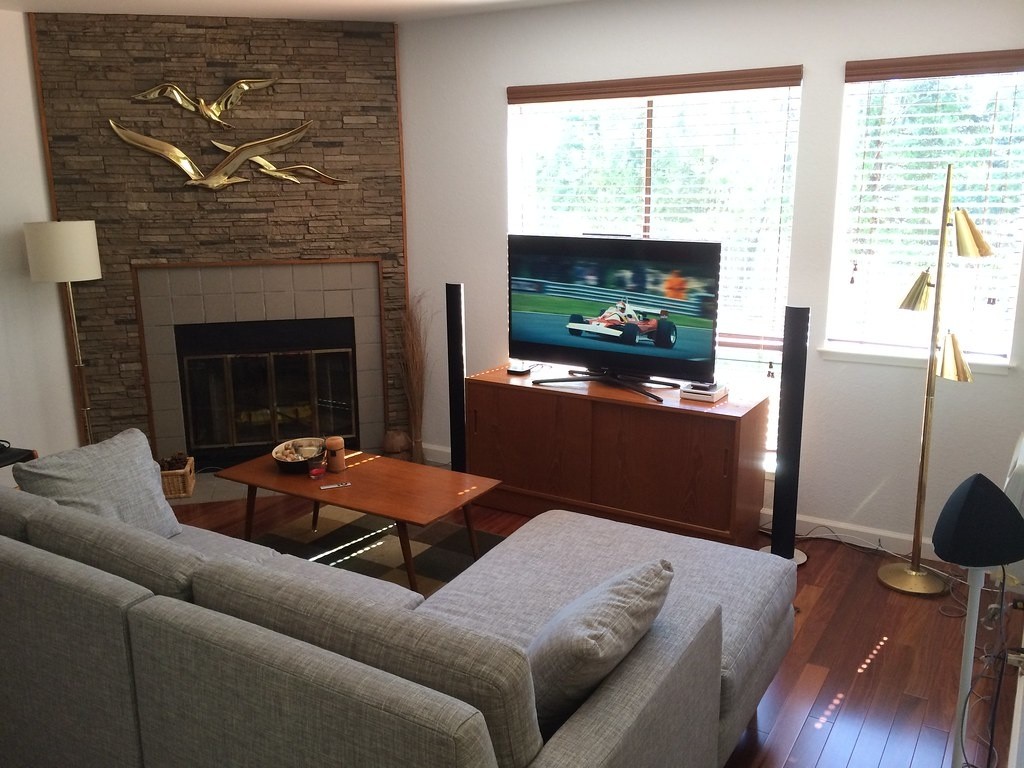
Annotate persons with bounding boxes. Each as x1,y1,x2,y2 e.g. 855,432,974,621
665,269,687,300
615,301,626,314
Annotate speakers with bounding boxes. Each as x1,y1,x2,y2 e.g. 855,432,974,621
445,282,466,472
759,305,810,564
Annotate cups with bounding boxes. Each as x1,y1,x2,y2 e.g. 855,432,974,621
308,457,325,479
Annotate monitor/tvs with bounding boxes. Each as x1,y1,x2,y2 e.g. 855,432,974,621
508,234,720,402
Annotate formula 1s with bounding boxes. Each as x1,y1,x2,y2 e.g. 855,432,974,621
568,297,678,350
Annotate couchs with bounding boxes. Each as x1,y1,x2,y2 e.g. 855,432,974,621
0,426,800,768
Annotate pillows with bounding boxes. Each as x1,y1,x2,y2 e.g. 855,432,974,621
525,558,675,745
11,428,183,538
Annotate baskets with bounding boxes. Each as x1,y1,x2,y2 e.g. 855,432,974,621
161,457,196,498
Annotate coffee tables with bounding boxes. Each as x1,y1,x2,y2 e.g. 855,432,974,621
213,448,502,596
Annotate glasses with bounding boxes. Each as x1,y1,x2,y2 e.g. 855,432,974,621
616,306,625,310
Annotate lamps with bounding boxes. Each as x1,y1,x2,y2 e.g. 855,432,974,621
876,163,994,599
24,219,102,447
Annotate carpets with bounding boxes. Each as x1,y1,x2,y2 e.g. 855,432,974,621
249,505,506,598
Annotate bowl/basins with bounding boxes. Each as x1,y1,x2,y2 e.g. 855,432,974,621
272,437,326,475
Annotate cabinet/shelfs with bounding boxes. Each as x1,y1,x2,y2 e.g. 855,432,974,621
463,361,771,549
